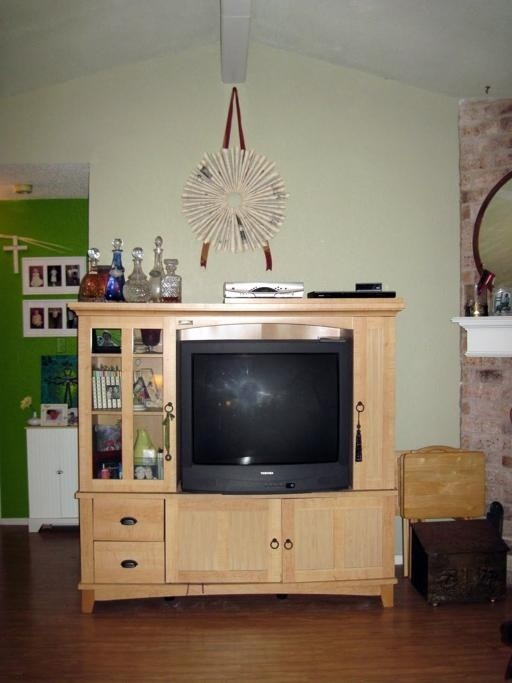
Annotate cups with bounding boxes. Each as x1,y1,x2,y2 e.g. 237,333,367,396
94,461,122,479
135,339,150,354
465,283,487,316
134,404,145,409
490,287,512,315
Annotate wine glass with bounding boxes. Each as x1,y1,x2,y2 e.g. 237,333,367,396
141,329,160,353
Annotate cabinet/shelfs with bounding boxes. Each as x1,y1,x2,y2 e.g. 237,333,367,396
68,295,406,613
24,427,81,534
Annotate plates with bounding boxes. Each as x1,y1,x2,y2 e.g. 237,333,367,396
133,408,146,410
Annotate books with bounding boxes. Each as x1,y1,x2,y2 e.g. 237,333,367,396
92,369,121,410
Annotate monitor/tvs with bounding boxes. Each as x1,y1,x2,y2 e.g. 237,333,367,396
177,339,353,493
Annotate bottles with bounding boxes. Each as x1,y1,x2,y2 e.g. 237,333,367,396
79,233,182,304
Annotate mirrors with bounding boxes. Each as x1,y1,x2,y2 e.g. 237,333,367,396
471,169,512,294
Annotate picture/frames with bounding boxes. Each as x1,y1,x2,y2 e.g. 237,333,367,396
40,403,69,427
19,254,88,339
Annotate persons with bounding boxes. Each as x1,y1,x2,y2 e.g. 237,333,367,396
31,309,43,327
136,466,145,479
147,467,155,479
67,265,78,276
68,310,76,328
69,271,80,285
47,409,61,418
102,331,114,345
52,308,60,327
51,267,61,286
31,266,43,286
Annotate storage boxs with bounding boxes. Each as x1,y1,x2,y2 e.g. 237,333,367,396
406,518,511,607
